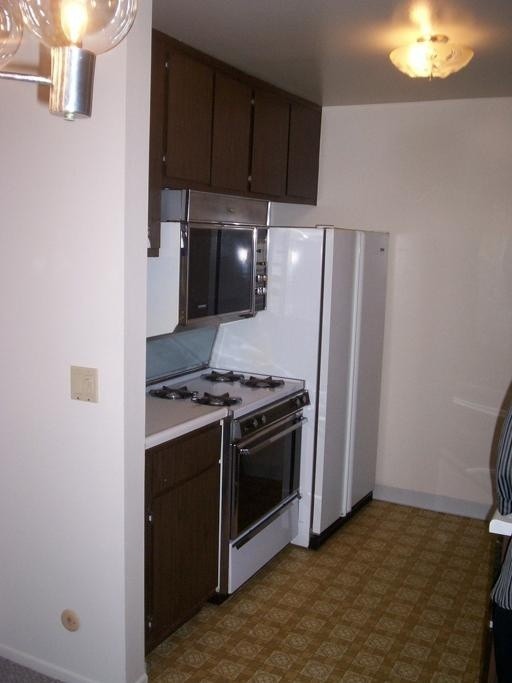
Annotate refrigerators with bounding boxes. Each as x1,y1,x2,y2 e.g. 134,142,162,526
212,226,388,550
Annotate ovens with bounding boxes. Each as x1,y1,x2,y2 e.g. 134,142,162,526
223,409,309,602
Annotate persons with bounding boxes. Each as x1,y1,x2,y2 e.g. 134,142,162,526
487,409,512,683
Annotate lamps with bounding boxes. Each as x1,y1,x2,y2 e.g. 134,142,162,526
390,36,475,81
0,1,140,124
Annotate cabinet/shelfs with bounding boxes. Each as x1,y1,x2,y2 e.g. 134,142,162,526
145,419,222,655
147,28,322,260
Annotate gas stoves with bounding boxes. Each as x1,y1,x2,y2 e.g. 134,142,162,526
149,362,310,442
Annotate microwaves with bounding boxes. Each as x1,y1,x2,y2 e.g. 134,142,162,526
146,187,268,342
